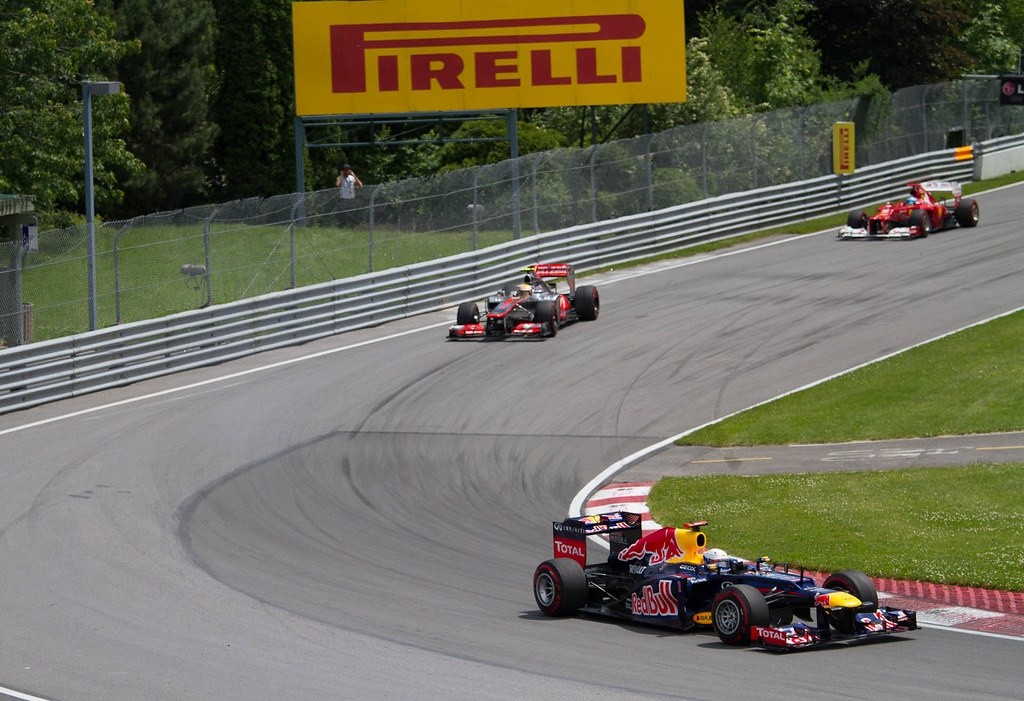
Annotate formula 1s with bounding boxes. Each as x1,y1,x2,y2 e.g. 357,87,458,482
445,263,600,342
836,180,978,242
533,508,920,654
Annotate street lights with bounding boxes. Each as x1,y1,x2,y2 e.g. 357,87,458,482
81,79,119,334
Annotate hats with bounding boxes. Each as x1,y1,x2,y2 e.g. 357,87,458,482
341,163,351,171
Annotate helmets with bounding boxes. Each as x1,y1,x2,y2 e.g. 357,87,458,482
519,284,532,292
907,197,918,202
703,548,731,573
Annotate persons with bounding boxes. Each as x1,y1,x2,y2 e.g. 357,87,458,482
906,187,925,205
703,549,730,573
517,284,531,300
335,164,363,229
